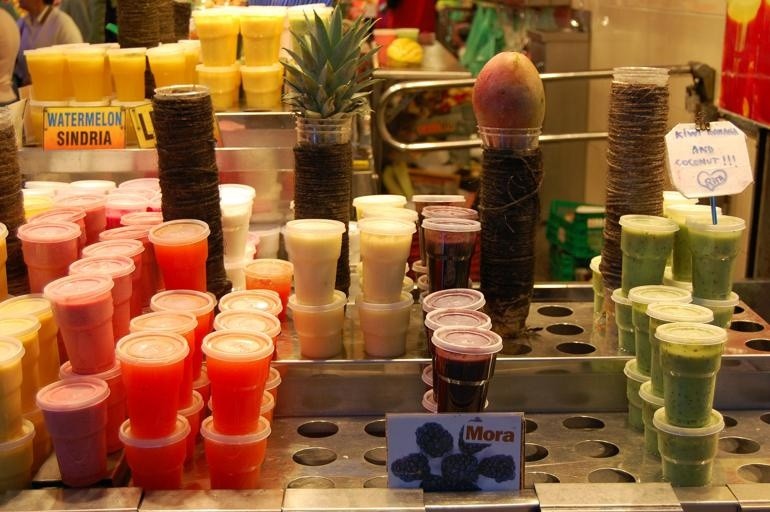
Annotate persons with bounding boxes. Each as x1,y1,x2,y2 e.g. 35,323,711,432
374,1,436,31
13,0,82,87
0,7,21,105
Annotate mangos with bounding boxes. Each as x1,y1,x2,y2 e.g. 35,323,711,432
471,51,547,150
